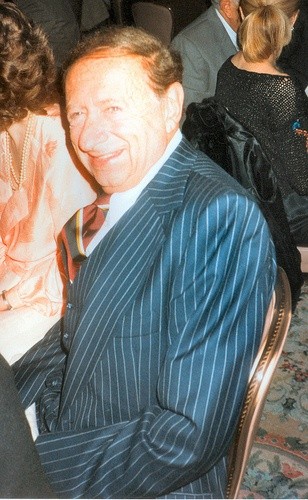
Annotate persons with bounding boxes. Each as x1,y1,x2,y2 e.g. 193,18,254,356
8,28,278,499
12,0,82,69
0,0,102,367
0,355,59,499
172,0,308,247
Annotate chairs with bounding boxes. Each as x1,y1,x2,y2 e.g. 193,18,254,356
185,99,303,310
130,0,176,52
224,264,293,499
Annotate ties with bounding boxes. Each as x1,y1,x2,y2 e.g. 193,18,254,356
60,193,111,317
